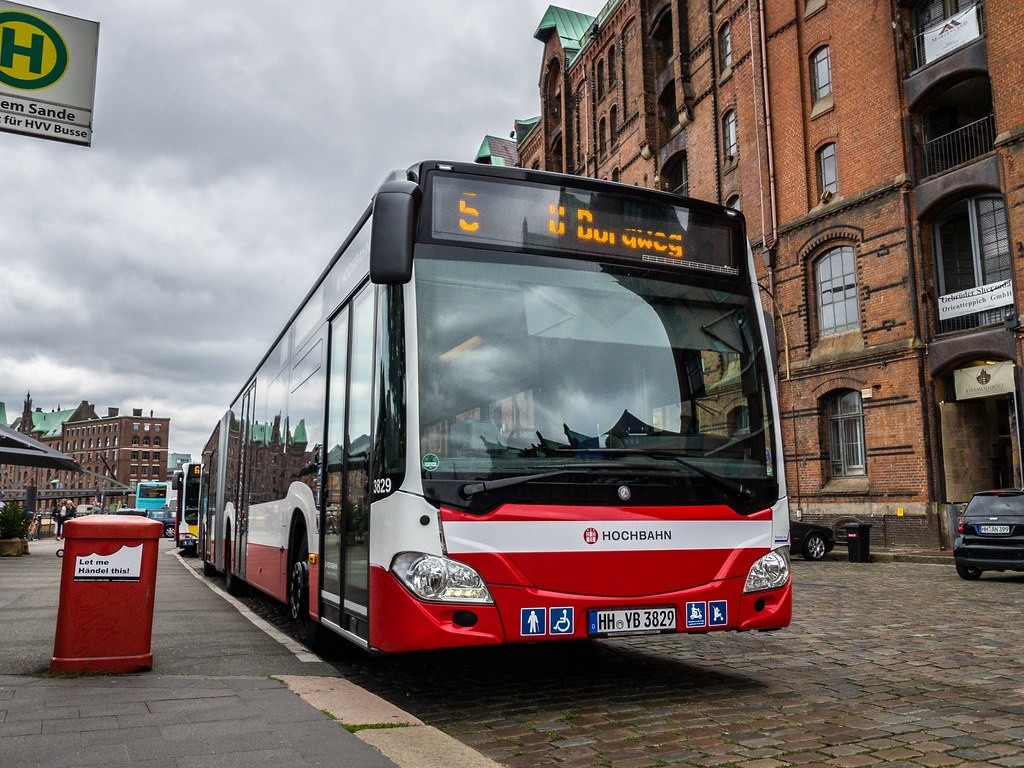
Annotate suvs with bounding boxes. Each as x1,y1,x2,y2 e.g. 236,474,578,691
953,488,1024,581
147,510,178,538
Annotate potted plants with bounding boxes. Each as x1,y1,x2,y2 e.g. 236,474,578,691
0,498,32,556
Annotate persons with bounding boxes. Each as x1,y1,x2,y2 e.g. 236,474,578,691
51,499,76,540
161,503,169,509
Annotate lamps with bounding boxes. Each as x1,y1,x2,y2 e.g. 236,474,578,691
510,131,517,139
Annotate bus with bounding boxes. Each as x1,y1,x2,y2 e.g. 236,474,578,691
135,480,178,509
198,161,791,655
172,462,201,552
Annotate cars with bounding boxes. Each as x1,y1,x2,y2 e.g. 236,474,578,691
789,517,837,562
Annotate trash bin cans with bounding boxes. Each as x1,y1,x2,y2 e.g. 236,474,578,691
845,522,872,563
116,508,149,518
49,515,163,678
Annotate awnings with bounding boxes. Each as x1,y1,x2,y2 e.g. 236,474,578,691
0,422,83,474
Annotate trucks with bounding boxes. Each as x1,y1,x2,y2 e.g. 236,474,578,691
169,497,193,518
